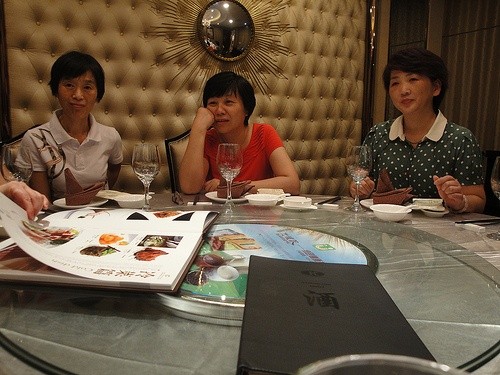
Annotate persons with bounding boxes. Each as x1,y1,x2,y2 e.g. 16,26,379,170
178,71,301,195
349,48,486,213
0,164,50,220
14,50,124,198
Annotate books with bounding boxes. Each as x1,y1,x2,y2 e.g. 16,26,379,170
0,192,220,295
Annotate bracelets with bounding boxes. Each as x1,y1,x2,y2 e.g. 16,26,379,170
445,195,468,213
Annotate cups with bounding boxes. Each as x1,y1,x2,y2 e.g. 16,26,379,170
194,253,250,282
2,146,34,183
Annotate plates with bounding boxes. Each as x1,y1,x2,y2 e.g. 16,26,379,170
360,199,412,209
421,209,449,217
205,191,248,203
52,198,108,209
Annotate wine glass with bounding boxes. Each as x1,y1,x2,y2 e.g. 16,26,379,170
131,144,161,212
344,146,372,214
216,144,244,208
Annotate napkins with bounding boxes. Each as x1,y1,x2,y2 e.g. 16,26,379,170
372,166,415,205
216,177,255,198
64,168,105,206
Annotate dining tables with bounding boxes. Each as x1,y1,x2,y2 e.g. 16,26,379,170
0,192,500,375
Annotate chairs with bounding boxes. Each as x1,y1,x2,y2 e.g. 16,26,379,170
164,129,192,192
1,123,42,175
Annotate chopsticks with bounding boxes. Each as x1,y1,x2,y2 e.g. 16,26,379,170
454,219,500,224
318,196,341,205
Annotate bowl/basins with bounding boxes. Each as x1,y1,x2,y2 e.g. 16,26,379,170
370,204,411,221
245,194,283,207
114,194,153,208
